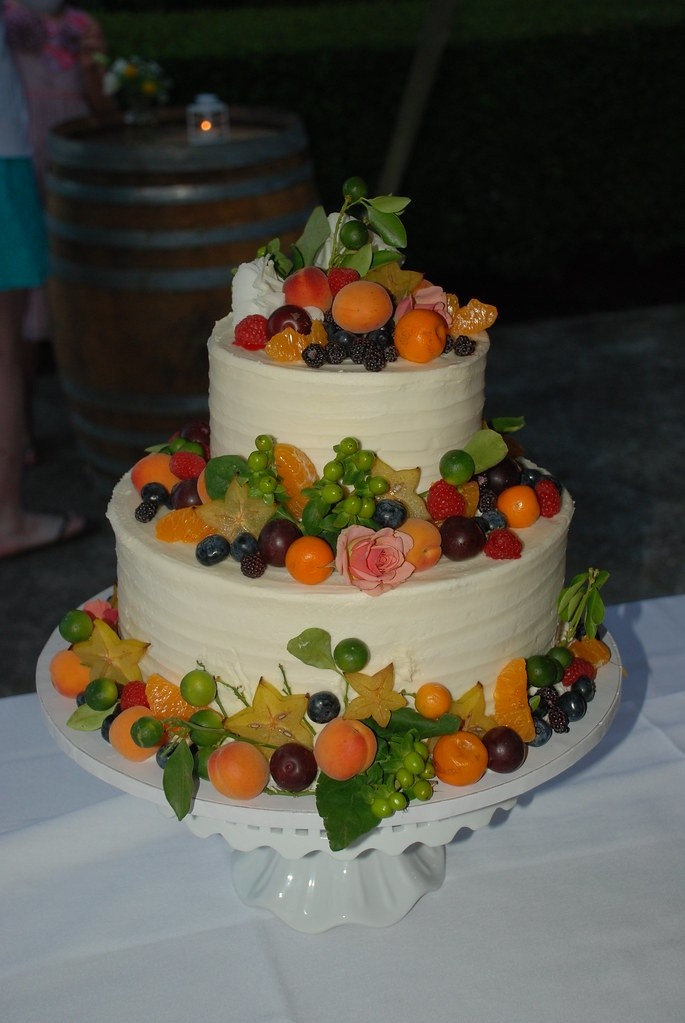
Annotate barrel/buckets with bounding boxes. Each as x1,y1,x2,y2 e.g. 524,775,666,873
47,106,319,539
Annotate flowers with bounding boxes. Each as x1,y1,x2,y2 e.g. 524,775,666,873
392,286,453,328
317,525,415,596
292,178,410,277
231,238,292,325
102,55,172,108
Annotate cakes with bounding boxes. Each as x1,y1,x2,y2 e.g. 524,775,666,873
37,181,609,850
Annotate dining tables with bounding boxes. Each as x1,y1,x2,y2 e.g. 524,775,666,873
0,591,684,1023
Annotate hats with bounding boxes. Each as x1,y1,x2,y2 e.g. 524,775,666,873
62,7,104,44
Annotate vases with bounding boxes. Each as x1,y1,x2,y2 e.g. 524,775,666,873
123,95,159,126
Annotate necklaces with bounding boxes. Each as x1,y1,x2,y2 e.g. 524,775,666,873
0,0,98,567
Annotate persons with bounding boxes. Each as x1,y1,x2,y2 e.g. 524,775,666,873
5,0,109,468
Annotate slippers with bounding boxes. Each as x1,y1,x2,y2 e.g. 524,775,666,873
1,511,98,562
